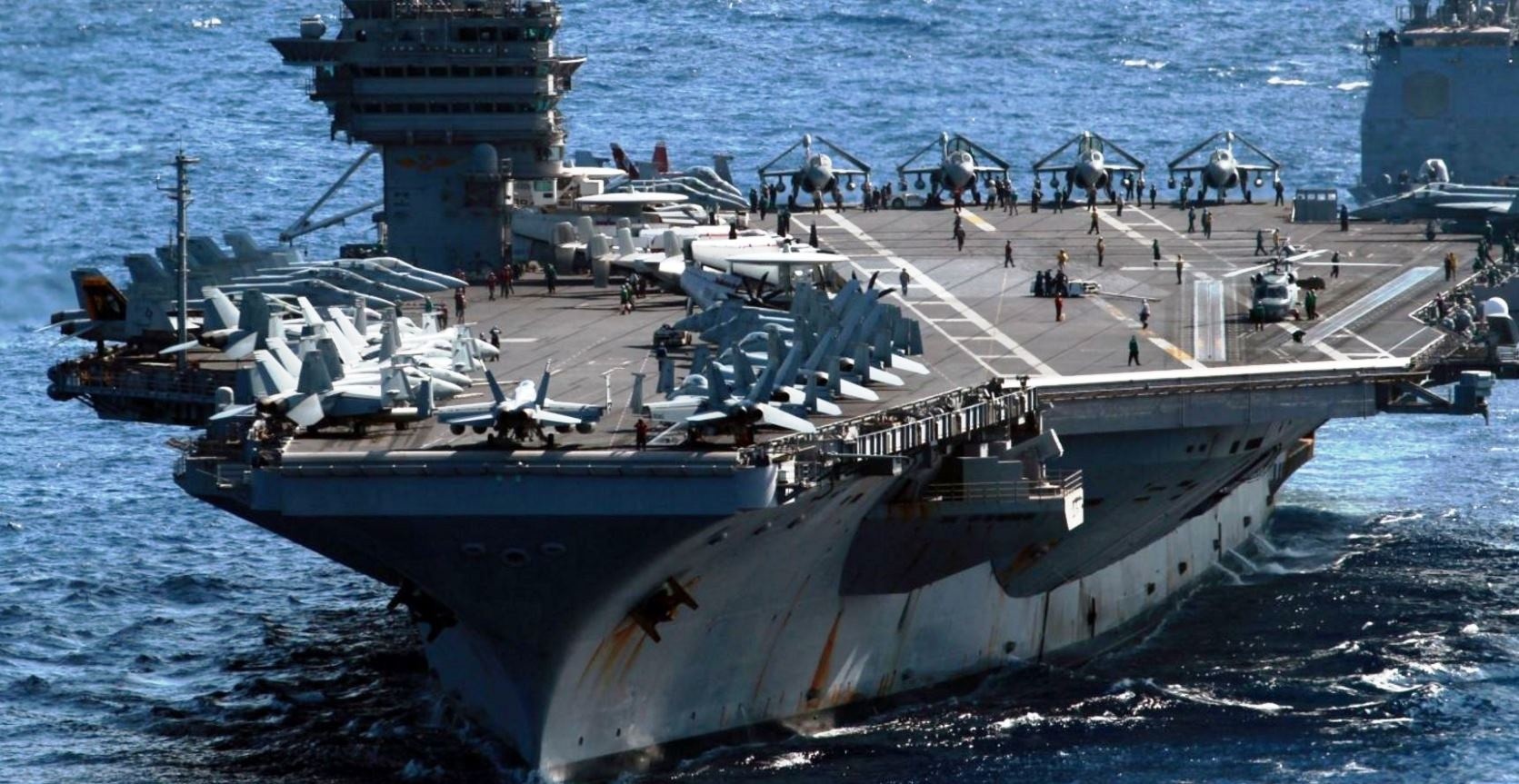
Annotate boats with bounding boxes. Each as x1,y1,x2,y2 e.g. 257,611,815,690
539,56,587,78
45,0,1519,783
267,17,357,62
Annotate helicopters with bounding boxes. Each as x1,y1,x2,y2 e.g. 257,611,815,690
1221,249,1403,321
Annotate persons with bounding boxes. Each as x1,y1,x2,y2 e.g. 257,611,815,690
394,262,558,362
618,271,647,315
1088,207,1105,267
1253,227,1281,257
1034,247,1070,321
656,341,668,371
1434,220,1519,333
1330,250,1339,279
1004,240,1015,268
952,189,966,251
634,418,646,451
1153,206,1212,285
1339,203,1349,231
1275,182,1285,206
1128,298,1151,367
749,181,893,248
1306,289,1320,320
983,172,1157,217
899,268,910,296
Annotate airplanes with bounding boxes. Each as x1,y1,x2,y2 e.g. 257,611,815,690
628,264,930,434
604,142,751,211
1033,131,1146,204
598,226,849,285
431,359,611,448
1348,182,1519,242
1167,130,1281,204
35,230,470,354
209,296,502,439
897,132,1011,209
756,134,871,210
